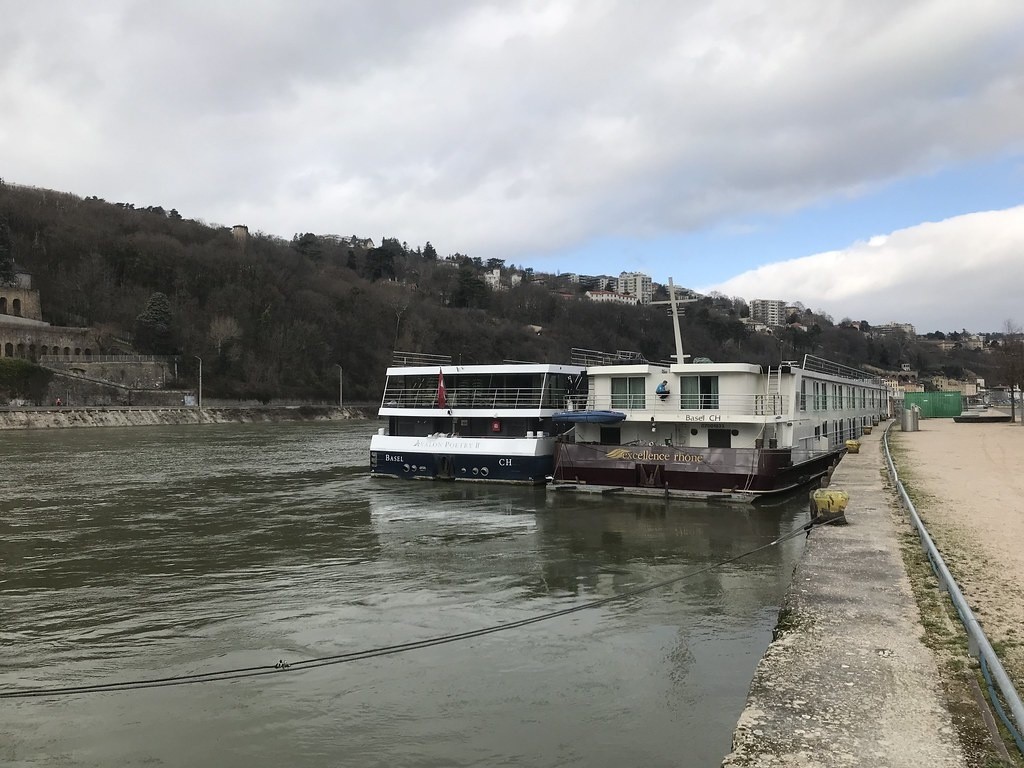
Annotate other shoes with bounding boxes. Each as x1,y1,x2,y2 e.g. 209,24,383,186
660,397,665,401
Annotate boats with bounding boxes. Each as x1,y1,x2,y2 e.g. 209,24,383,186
552,411,627,424
370,351,589,486
552,274,892,504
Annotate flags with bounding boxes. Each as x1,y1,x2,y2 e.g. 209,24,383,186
438,369,445,409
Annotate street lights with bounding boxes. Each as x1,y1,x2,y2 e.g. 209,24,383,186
195,355,201,406
335,365,342,405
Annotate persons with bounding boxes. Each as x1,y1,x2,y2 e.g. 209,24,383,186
567,375,575,410
656,380,670,401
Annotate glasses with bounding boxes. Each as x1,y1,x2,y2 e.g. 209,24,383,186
665,384,666,385
